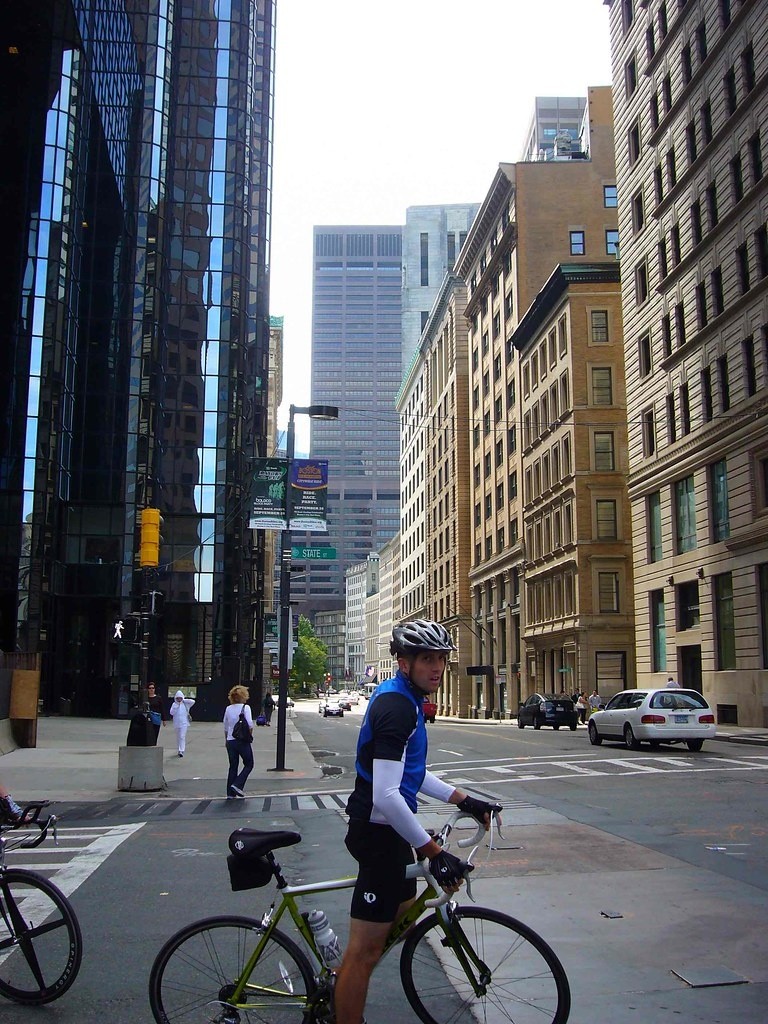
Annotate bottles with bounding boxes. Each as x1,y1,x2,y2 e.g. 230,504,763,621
308,909,344,969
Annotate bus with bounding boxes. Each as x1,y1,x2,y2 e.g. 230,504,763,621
365,683,379,700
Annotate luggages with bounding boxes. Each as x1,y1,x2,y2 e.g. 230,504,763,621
256,712,266,726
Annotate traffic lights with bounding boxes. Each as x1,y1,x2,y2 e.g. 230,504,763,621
328,678,330,680
110,617,139,643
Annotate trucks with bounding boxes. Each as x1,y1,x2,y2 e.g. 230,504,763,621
359,689,365,696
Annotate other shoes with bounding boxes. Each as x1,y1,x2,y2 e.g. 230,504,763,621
178,752,184,756
230,785,245,797
227,795,234,800
266,724,270,726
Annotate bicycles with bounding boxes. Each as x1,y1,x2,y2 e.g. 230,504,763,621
0,799,84,1005
148,795,573,1024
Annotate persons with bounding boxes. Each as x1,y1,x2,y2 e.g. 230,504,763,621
170,690,195,757
223,685,254,800
144,682,166,746
332,617,502,1024
0,785,31,828
560,688,601,725
263,693,275,727
666,677,680,688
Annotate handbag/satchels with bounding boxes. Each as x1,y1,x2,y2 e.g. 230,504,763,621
127,710,161,747
575,696,586,709
232,704,253,746
183,701,192,722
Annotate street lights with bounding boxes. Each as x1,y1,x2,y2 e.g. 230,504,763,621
323,672,330,697
273,401,339,769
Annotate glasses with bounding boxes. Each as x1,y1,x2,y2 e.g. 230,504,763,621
149,687,154,690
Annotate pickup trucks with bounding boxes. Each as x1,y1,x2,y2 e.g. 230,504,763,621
421,697,437,723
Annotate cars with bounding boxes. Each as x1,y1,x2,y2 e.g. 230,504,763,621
318,688,365,705
318,700,343,718
272,695,279,706
337,699,351,711
588,688,716,751
517,692,579,730
286,697,294,708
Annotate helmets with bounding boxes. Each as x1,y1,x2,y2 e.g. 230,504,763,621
389,619,458,652
